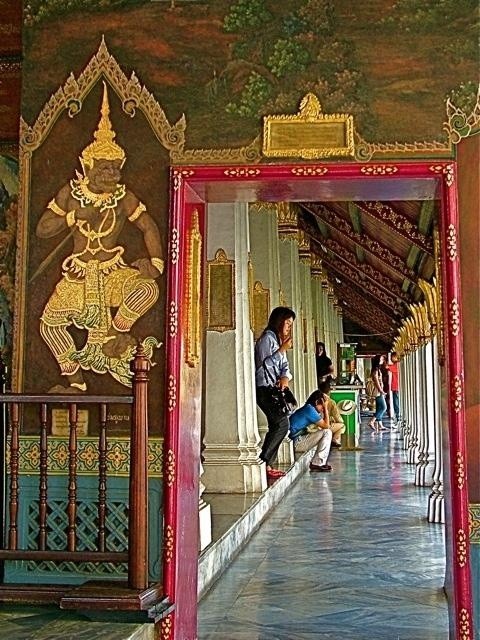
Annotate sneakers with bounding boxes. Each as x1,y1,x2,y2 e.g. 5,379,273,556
331,441,341,447
310,462,332,472
267,470,285,479
367,422,376,430
378,427,388,430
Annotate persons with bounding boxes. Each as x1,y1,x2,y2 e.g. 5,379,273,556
367,353,389,432
34,139,165,394
383,352,400,429
320,381,346,450
316,341,335,396
254,306,296,479
287,389,333,472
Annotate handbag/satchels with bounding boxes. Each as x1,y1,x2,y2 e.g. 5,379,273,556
366,382,380,397
268,385,297,415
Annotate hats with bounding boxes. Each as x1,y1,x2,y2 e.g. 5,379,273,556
336,400,356,415
